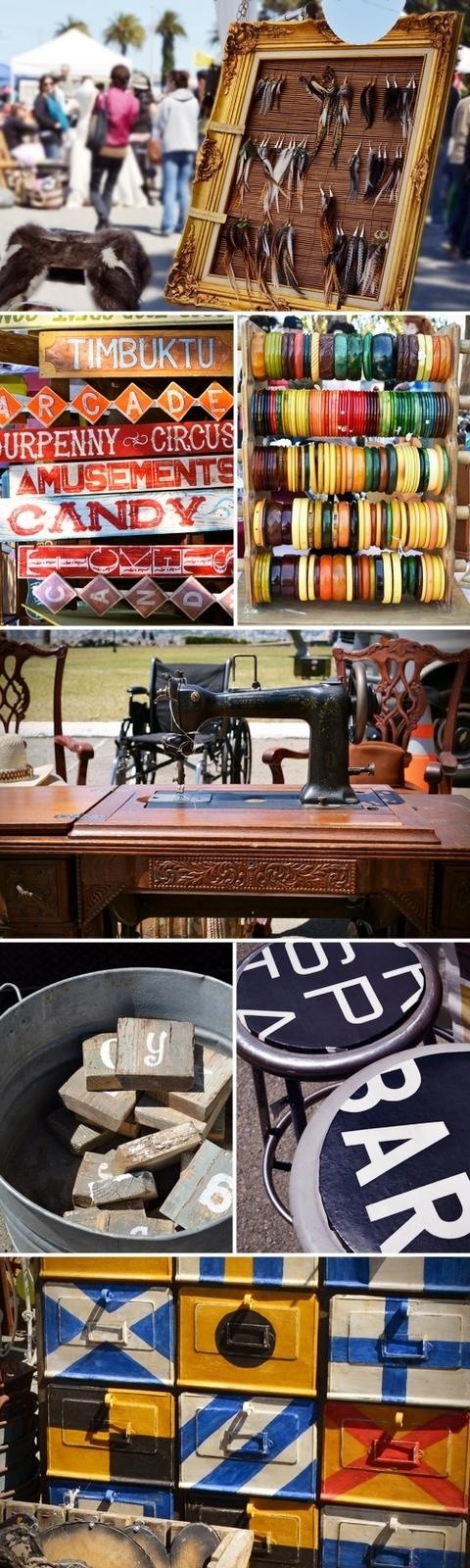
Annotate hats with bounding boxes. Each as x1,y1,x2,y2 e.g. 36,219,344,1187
0,733,55,787
131,75,150,90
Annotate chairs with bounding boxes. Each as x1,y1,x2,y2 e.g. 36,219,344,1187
0,629,94,787
261,638,470,794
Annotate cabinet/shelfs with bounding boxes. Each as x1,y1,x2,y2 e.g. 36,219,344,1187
239,318,470,613
34,1257,470,1567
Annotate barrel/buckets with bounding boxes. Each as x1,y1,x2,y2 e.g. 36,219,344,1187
0,966,232,1252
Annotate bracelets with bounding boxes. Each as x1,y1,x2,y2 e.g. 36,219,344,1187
249,331,453,606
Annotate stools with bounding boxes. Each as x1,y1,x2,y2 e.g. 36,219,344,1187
230,937,445,1228
287,1039,469,1257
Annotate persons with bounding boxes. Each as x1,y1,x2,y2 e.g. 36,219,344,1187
0,63,203,237
425,71,470,263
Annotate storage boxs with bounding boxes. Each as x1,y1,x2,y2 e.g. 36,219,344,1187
1,1500,257,1567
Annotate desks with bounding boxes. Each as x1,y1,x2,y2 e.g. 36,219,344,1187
0,786,469,982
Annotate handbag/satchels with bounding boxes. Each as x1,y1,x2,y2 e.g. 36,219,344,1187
349,741,411,788
147,141,161,165
85,110,108,154
23,172,64,209
